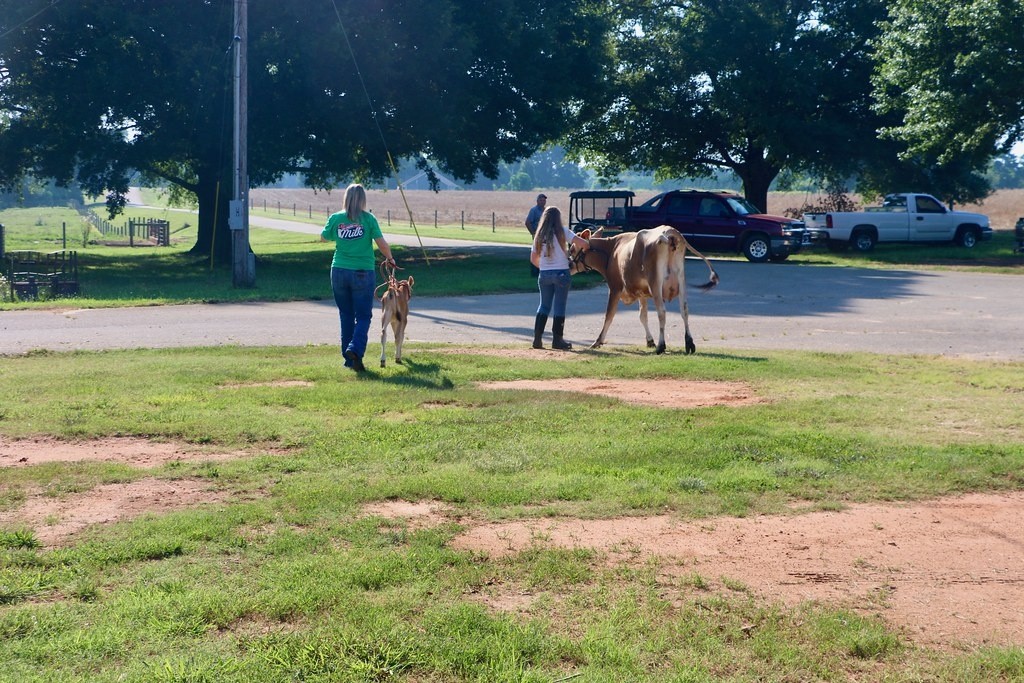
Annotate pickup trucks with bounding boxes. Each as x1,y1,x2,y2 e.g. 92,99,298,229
799,192,994,253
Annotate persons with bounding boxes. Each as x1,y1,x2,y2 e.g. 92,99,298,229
525,194,547,278
321,182,394,371
530,206,589,350
1015,218,1024,253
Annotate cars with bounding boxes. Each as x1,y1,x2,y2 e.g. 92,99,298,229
605,189,813,264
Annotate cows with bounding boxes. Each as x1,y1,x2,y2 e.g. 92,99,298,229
569,225,720,356
379,275,415,367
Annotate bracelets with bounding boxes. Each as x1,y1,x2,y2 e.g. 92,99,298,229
386,257,393,262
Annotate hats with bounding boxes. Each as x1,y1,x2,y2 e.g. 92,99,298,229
537,194,547,201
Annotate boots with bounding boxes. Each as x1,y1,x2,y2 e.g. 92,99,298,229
533,314,548,347
552,315,572,348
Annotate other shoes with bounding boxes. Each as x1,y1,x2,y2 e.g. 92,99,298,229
343,348,365,372
